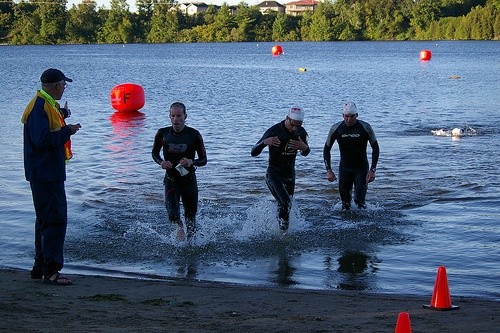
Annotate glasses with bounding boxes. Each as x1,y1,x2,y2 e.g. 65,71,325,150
289,118,301,128
345,114,355,118
58,83,67,88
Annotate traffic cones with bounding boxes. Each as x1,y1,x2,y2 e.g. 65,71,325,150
394,311,412,333
422,266,460,312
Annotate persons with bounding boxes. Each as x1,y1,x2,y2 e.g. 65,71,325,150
251,106,311,236
152,103,207,240
22,68,81,286
323,101,380,212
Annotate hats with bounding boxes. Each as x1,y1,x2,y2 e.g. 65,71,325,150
41,68,73,83
343,101,357,114
288,107,305,121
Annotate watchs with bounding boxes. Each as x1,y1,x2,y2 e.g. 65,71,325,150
371,168,376,173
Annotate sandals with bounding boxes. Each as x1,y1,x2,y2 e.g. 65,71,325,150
42,272,74,286
31,272,42,278
